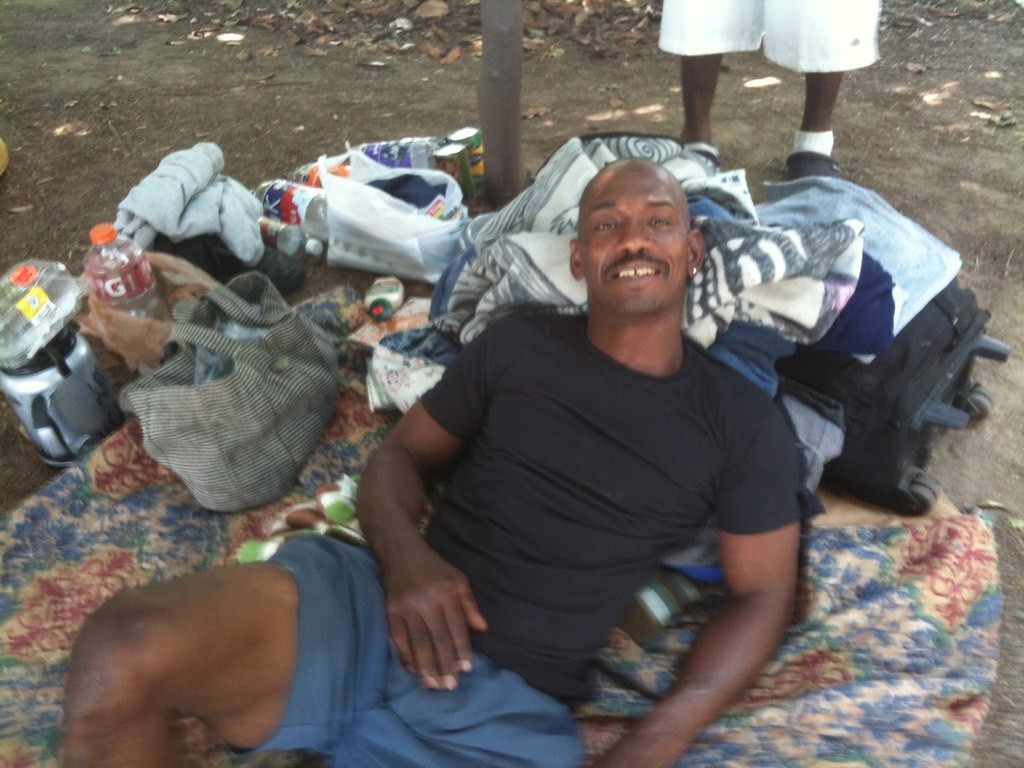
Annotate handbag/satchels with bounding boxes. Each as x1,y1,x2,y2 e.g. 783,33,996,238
88,249,341,513
316,141,470,284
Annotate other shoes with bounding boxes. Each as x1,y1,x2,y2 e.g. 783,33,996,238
786,151,842,181
682,140,723,167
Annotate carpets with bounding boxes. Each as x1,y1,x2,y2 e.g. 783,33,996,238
0,297,1001,768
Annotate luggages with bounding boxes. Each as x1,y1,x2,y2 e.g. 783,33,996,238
786,277,1013,518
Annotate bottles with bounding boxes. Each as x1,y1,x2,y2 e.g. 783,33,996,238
84,222,175,369
244,125,485,259
364,275,404,320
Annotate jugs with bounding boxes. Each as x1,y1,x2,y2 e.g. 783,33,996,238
0,321,123,467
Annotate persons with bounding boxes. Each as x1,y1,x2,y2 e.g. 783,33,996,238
658,0,885,180
62,159,826,768
